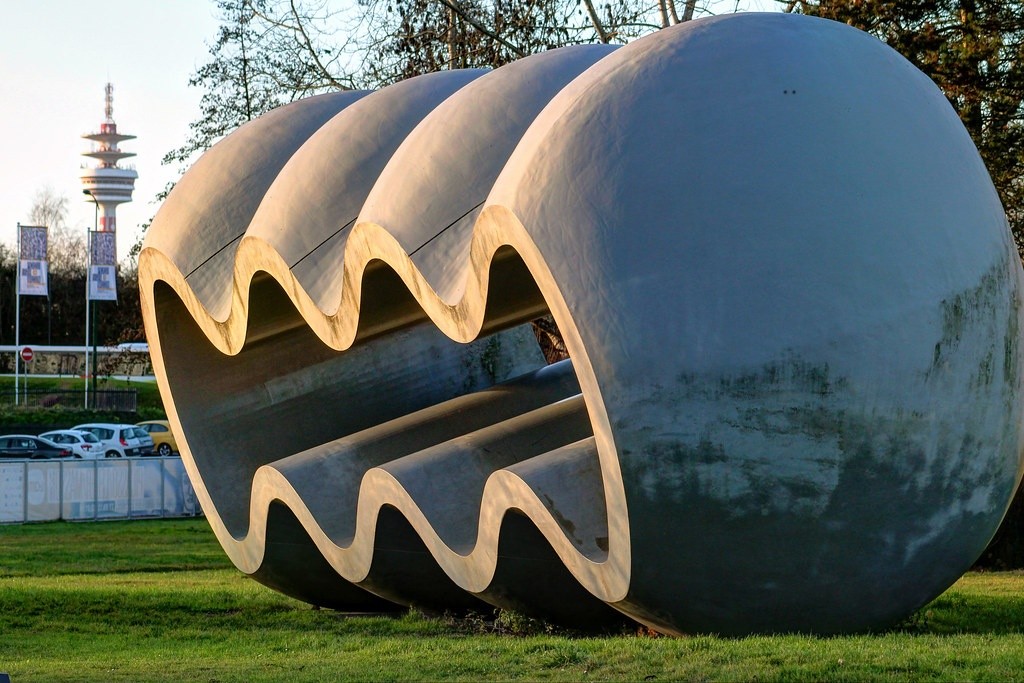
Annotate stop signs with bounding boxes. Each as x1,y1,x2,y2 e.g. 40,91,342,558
21,348,35,362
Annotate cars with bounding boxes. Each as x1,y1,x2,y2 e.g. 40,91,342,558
38,430,105,459
0,435,76,462
135,418,180,457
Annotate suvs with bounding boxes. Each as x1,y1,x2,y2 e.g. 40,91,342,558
71,423,156,458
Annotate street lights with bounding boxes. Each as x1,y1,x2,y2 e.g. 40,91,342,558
81,188,100,410
